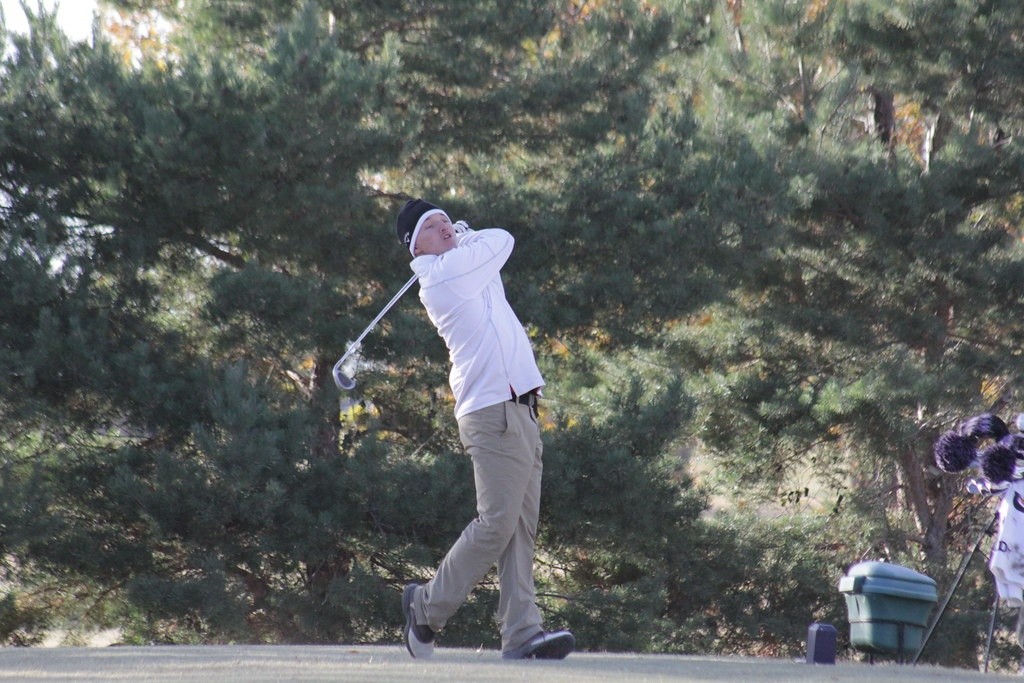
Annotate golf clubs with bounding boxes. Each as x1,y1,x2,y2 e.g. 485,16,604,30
331,220,469,391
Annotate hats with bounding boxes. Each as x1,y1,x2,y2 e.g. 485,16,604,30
396,200,451,259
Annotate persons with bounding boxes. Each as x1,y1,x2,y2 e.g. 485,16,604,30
394,199,577,661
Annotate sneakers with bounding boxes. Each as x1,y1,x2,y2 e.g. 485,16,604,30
402,583,434,659
504,629,574,660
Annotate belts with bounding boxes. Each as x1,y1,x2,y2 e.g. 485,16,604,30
509,393,536,409
